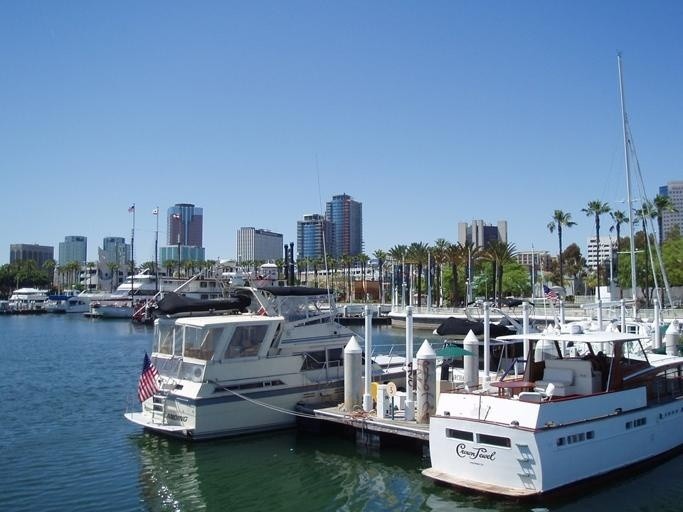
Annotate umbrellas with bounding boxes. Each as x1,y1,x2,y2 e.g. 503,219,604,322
430,344,476,382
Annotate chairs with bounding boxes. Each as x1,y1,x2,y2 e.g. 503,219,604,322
519,392,541,404
482,376,503,394
539,382,555,402
464,384,486,395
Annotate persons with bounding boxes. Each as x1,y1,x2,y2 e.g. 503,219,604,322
592,350,609,372
435,359,451,381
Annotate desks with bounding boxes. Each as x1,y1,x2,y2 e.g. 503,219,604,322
489,381,536,398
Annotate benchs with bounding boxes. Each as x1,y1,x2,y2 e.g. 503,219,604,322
544,359,602,394
533,368,577,397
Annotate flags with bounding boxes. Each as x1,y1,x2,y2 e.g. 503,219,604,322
126,205,134,214
136,355,160,403
150,208,158,216
542,283,559,303
